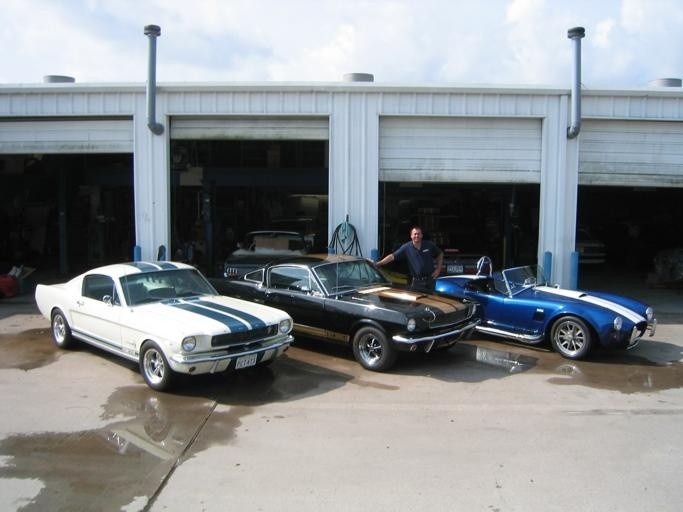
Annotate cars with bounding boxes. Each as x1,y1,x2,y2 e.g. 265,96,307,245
230,230,309,259
34,260,293,392
222,254,483,372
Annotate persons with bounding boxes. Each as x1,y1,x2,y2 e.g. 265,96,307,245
367,225,444,292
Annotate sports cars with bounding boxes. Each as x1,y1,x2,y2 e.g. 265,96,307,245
434,256,658,361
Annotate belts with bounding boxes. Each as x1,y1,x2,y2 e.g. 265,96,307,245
412,277,432,281
